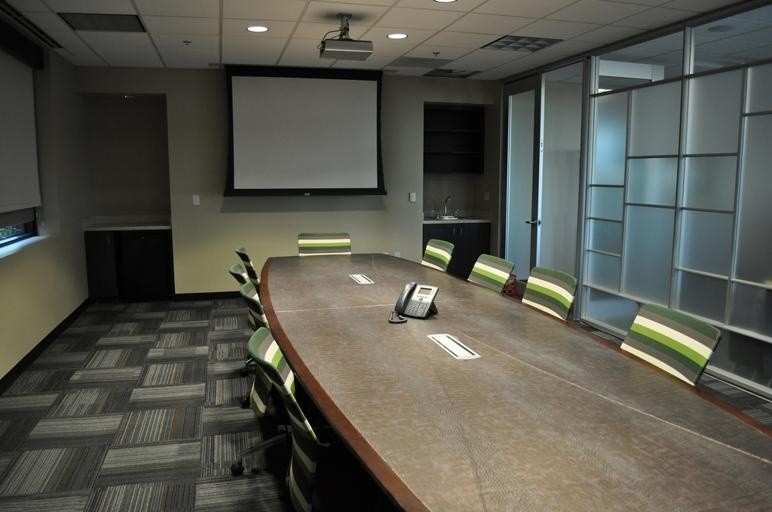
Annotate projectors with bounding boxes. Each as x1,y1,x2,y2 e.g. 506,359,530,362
320,38,374,60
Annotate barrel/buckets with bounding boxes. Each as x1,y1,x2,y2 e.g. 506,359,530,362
503,274,516,295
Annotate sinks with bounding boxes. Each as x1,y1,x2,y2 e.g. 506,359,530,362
438,217,458,220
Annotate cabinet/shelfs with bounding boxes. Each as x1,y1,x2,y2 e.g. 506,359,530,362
84,229,176,304
423,223,493,284
423,104,486,175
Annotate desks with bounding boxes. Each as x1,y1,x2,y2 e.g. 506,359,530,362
261,250,771,512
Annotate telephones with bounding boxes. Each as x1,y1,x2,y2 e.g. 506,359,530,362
395,282,439,318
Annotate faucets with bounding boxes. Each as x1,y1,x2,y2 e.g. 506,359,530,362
444,196,452,216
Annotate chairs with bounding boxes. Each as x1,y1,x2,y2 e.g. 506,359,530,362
228,248,292,476
521,267,578,322
247,326,372,511
467,253,515,295
298,233,352,257
620,302,722,387
421,238,454,273
